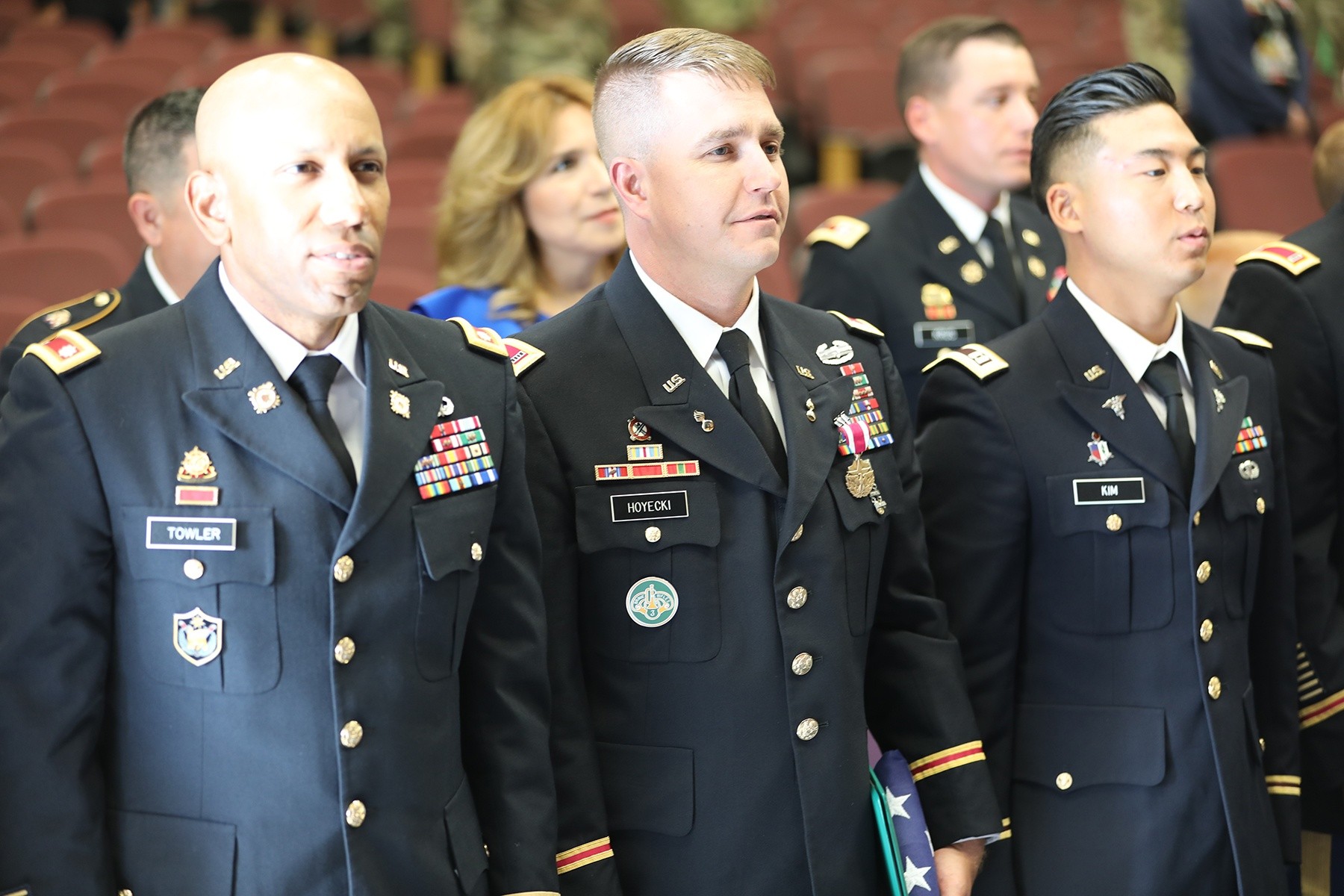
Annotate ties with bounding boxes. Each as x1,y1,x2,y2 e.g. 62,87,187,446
715,326,789,487
286,355,358,489
981,217,1023,308
1141,353,1195,488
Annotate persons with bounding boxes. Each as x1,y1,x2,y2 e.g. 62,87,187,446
1309,120,1344,216
1,49,559,896
1185,0,1315,146
911,61,1305,894
794,11,1066,392
0,86,221,399
1214,211,1344,896
505,21,1017,896
407,74,625,337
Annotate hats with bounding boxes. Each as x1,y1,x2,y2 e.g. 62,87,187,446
867,748,940,896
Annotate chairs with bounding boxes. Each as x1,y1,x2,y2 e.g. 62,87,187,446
0,0,1344,350
